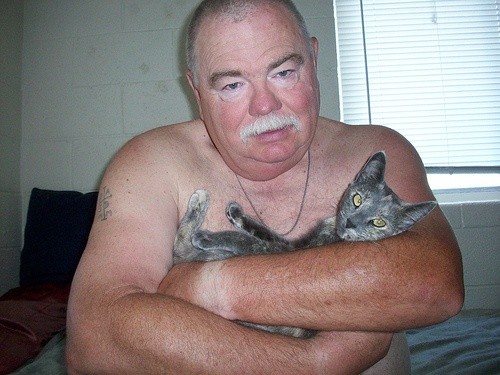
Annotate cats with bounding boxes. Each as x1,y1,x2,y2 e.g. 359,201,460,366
175,149,439,340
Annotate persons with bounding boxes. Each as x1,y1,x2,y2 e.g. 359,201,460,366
65,0,465,375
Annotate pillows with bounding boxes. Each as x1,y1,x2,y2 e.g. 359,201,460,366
18,188,97,287
0,283,72,375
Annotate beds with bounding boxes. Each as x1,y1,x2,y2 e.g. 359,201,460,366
12,308,500,375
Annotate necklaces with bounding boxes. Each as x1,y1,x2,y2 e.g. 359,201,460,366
233,151,309,237
